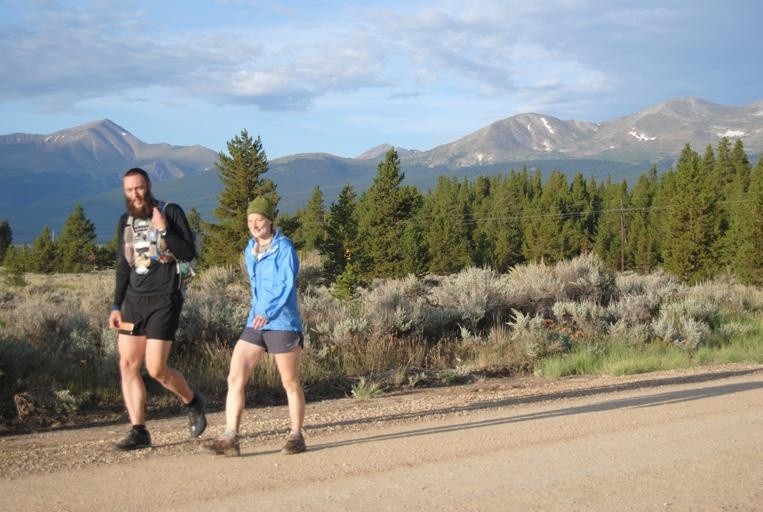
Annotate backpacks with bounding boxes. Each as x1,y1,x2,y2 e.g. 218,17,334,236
147,201,205,300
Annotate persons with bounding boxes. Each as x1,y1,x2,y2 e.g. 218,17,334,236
108,169,206,452
197,196,307,457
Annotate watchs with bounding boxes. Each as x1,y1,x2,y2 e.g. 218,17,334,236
157,227,168,238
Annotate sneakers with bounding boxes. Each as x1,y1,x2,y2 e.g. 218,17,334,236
193,435,240,457
115,428,152,451
183,392,206,438
281,433,306,455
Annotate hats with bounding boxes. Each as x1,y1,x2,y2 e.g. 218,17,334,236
246,196,275,222
